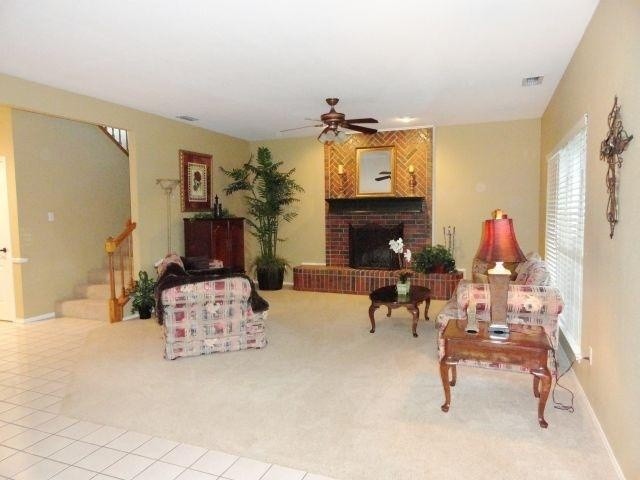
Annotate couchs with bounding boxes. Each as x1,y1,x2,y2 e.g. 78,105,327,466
436,251,564,377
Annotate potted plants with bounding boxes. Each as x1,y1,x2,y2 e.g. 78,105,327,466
410,245,454,274
225,145,305,292
126,270,156,319
396,272,412,295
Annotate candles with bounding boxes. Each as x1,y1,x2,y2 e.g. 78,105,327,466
338,165,344,174
409,165,414,173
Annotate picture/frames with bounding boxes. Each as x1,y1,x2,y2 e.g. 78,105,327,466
178,148,213,213
354,145,396,197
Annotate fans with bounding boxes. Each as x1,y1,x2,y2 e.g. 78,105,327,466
279,97,381,146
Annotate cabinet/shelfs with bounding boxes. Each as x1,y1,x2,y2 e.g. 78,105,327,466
183,216,246,274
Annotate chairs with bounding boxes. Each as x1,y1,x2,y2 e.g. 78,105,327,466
156,254,269,359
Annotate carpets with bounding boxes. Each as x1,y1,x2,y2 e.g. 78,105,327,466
60,286,623,478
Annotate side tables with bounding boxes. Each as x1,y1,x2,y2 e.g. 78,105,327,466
439,319,552,429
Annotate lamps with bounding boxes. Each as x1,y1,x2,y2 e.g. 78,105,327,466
317,126,348,146
474,208,527,333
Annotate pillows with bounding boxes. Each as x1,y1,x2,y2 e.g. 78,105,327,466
514,250,550,286
